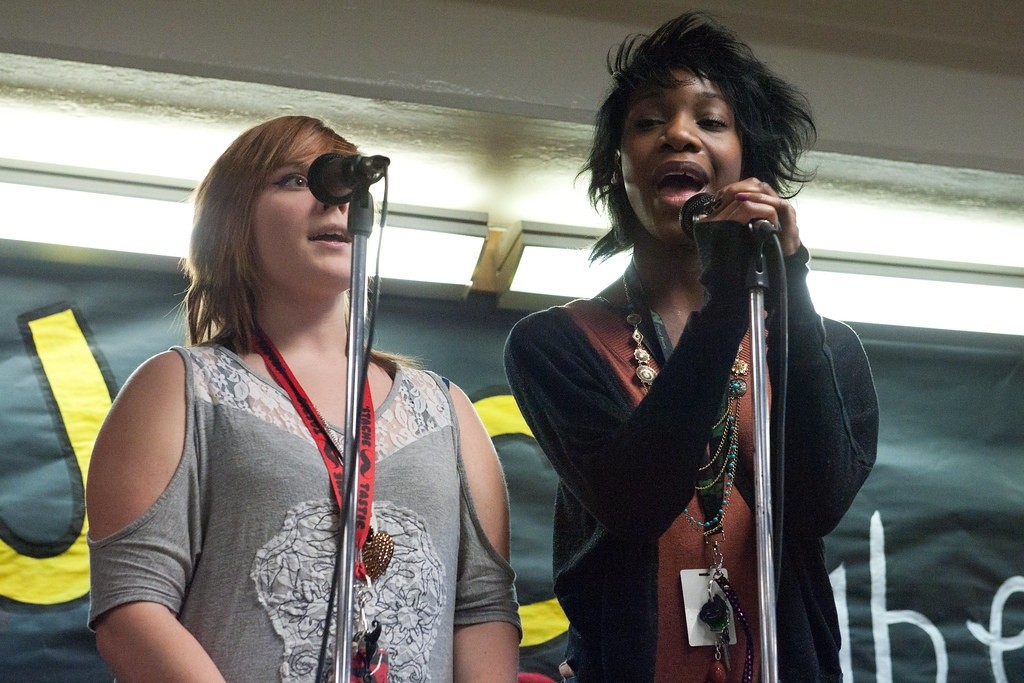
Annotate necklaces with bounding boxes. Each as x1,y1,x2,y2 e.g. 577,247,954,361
622,267,758,683
313,405,395,580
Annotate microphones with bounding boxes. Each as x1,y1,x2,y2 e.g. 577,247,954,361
307,152,390,205
679,192,778,244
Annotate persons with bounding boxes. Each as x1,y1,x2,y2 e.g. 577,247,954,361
85,115,522,683
502,8,876,683
